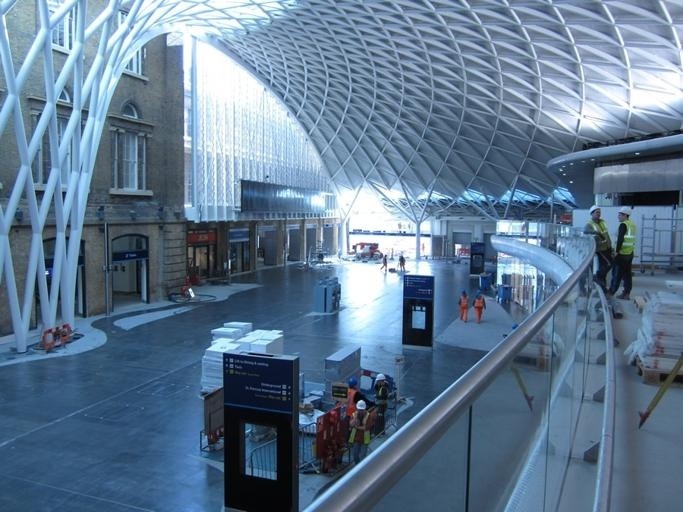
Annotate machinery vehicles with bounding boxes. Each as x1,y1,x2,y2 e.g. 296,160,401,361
349,242,383,260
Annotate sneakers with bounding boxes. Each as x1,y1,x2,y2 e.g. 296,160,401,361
616,290,630,301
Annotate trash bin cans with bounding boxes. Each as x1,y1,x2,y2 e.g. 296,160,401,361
497,284,512,305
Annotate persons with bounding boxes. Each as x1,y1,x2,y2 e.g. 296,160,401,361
583,205,613,294
372,373,389,413
608,206,636,300
382,255,387,272
44,323,68,354
347,399,372,465
398,255,405,272
458,290,469,323
347,377,372,436
473,294,486,323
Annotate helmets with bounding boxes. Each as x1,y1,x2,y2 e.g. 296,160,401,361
348,377,358,388
616,206,632,216
477,289,482,293
460,288,466,295
589,205,601,215
376,373,386,381
356,400,367,410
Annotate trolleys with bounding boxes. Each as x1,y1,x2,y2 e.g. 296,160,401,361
638,214,682,276
